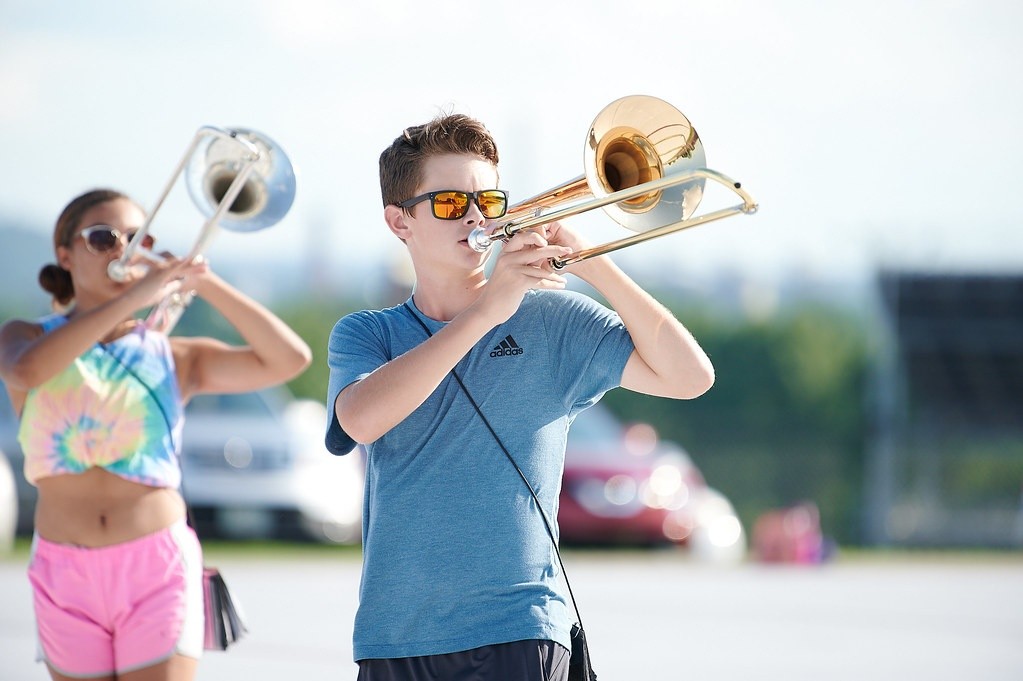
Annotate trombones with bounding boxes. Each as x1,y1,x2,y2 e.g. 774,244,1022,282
468,91,758,276
107,120,297,336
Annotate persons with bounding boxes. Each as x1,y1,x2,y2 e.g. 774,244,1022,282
0,187,311,681
325,113,715,681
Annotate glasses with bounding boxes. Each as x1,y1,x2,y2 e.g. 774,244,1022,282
397,189,509,221
72,224,155,256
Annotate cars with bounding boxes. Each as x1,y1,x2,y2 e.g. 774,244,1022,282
557,404,690,548
180,398,372,553
